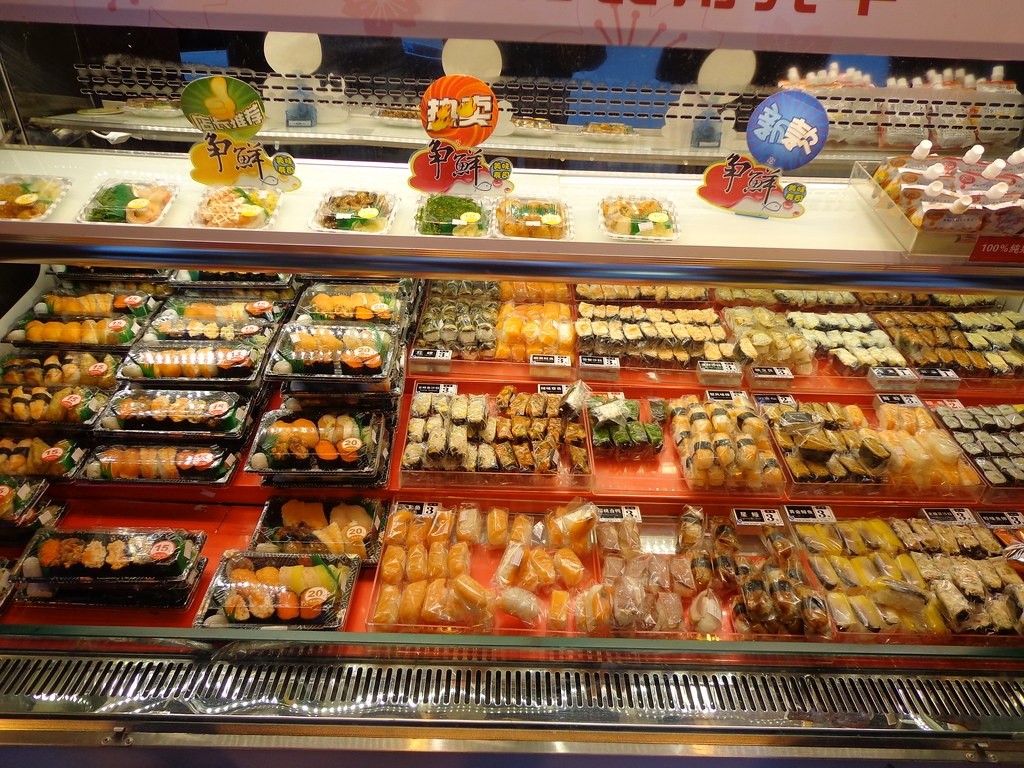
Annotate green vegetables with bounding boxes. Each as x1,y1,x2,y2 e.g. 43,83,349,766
419,194,487,234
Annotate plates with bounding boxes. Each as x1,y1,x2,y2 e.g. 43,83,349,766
77,109,124,114
579,128,640,141
131,107,183,117
369,109,421,127
514,125,558,136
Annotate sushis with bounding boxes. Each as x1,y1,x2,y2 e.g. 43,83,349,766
0,269,1024,639
